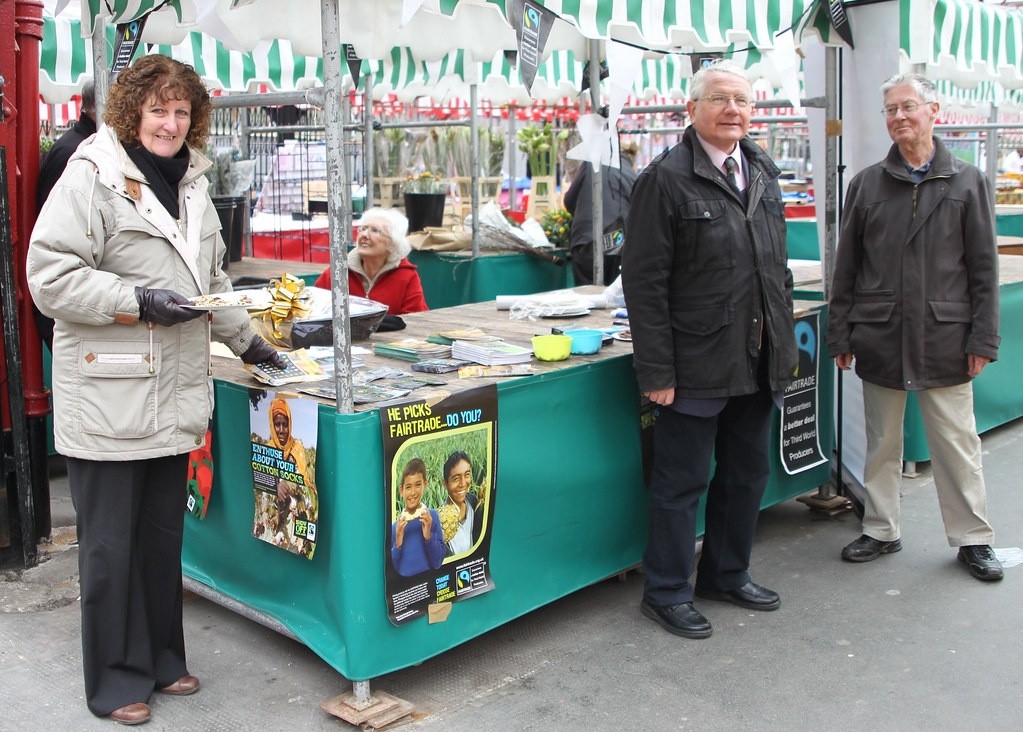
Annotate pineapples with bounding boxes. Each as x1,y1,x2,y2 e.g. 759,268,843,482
435,503,459,544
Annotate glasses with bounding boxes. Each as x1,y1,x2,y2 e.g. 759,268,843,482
694,94,749,107
881,101,934,120
357,226,393,239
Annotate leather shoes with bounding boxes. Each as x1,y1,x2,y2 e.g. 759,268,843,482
108,702,151,724
695,580,780,610
641,599,713,639
161,674,200,695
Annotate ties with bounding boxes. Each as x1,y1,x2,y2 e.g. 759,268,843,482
724,157,736,186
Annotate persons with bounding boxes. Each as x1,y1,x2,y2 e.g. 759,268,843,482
35,68,112,218
565,142,638,287
392,458,444,576
264,398,312,555
827,73,1003,582
440,452,485,557
26,54,287,725
620,60,799,639
315,207,430,316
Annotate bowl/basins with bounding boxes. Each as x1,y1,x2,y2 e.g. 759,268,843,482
563,330,604,355
532,335,573,361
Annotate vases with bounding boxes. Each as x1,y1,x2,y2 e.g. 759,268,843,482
229,196,246,264
406,191,447,232
211,197,234,270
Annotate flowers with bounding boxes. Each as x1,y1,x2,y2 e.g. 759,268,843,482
401,173,441,193
542,209,574,248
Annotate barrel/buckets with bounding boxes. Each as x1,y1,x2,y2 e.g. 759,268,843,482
212,196,247,263
404,193,445,232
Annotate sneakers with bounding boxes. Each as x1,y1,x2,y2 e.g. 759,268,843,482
842,535,902,561
958,545,1004,581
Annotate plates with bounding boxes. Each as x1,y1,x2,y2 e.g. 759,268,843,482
611,332,634,341
180,292,274,310
544,310,590,318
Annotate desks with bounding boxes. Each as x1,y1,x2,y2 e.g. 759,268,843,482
182,204,1022,712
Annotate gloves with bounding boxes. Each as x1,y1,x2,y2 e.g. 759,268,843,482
135,286,209,326
240,335,287,369
